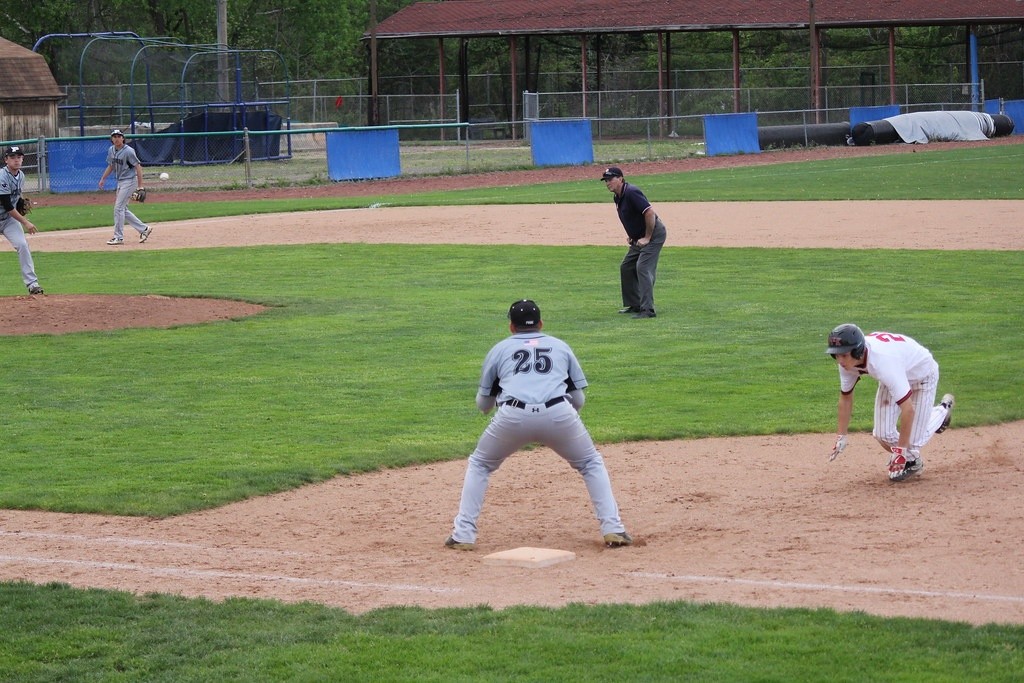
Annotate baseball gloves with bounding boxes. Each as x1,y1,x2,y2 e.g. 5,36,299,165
132,188,147,203
17,197,31,216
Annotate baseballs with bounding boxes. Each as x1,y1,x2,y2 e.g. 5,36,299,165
160,173,169,181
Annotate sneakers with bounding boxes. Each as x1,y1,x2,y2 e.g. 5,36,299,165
29,287,43,295
889,456,924,481
106,238,124,245
604,532,633,545
934,394,954,433
445,535,473,550
139,226,153,243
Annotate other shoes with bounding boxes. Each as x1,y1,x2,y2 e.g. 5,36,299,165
619,306,639,313
631,310,656,319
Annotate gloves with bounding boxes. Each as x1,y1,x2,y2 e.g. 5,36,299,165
886,446,907,479
829,434,846,462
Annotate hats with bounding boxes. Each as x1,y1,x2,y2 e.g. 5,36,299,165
600,168,623,181
4,146,24,157
508,299,540,326
111,129,124,136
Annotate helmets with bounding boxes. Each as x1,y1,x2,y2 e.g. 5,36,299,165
825,324,866,360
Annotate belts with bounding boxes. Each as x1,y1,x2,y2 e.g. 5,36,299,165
506,396,565,409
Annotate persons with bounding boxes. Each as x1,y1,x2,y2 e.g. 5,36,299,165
600,167,667,319
98,130,153,245
825,324,955,483
445,301,632,551
0,146,44,297
